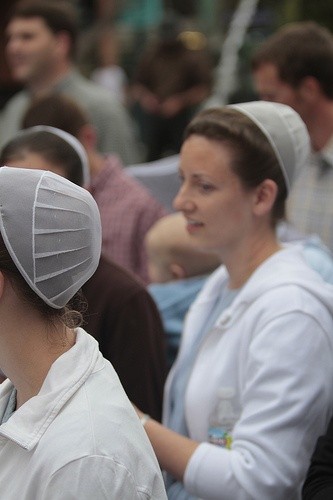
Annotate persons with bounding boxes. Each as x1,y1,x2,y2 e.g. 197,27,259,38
0,0,333,499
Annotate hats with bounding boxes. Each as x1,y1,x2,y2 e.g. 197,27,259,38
226,101,311,196
0,166,102,308
0,125,89,189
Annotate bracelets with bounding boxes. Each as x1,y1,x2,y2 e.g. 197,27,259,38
140,413,150,425
